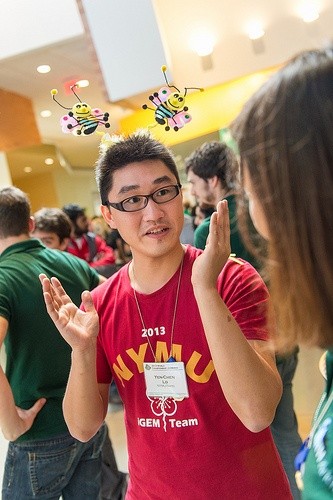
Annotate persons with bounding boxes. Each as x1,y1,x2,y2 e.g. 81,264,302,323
233,48,333,500
30,142,301,500
0,187,124,500
38,137,283,500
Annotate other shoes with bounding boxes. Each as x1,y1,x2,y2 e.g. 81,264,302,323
109,382,122,403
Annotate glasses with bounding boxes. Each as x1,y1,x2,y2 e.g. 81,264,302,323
107,184,182,212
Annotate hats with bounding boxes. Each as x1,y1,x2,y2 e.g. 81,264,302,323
63,204,85,217
